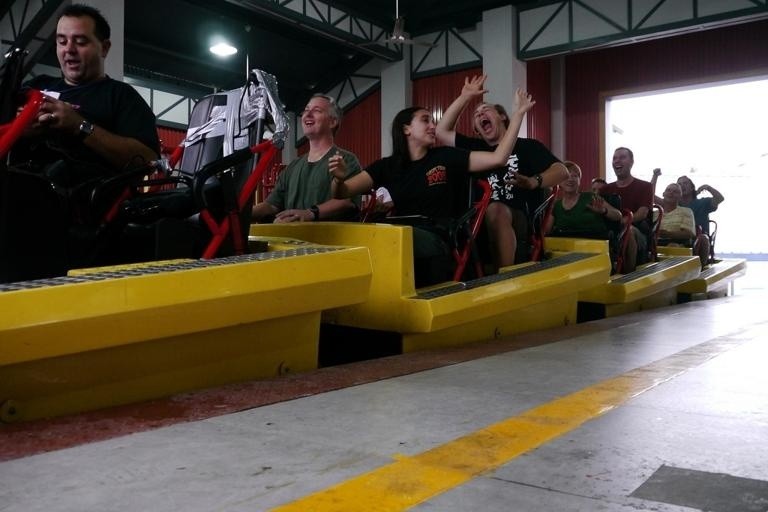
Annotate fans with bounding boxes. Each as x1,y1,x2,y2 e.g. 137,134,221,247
354,0,439,48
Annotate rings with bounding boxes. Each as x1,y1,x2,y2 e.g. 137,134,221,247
51,113,56,121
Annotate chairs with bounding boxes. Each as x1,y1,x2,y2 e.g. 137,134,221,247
709,221,718,259
452,179,490,282
615,208,634,272
530,183,560,263
652,204,664,254
694,227,702,254
101,82,287,263
359,188,377,224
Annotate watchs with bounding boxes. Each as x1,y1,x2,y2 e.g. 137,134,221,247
73,118,94,139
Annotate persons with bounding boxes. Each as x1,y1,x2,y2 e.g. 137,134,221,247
11,5,164,212
